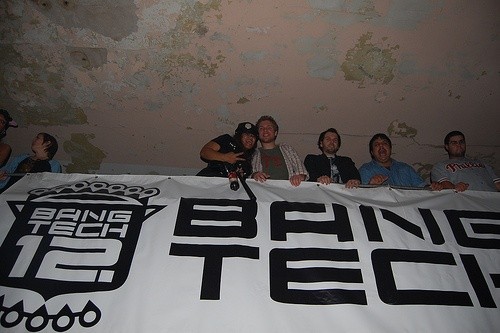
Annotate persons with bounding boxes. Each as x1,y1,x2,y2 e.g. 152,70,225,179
303,128,362,190
195,122,259,178
246,116,310,187
358,133,444,191
430,131,500,193
1,132,62,189
1,109,18,182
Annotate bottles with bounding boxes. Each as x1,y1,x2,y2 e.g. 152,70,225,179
228,169,239,191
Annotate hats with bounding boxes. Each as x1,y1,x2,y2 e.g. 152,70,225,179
235,122,258,135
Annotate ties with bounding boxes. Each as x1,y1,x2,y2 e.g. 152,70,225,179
328,157,339,183
